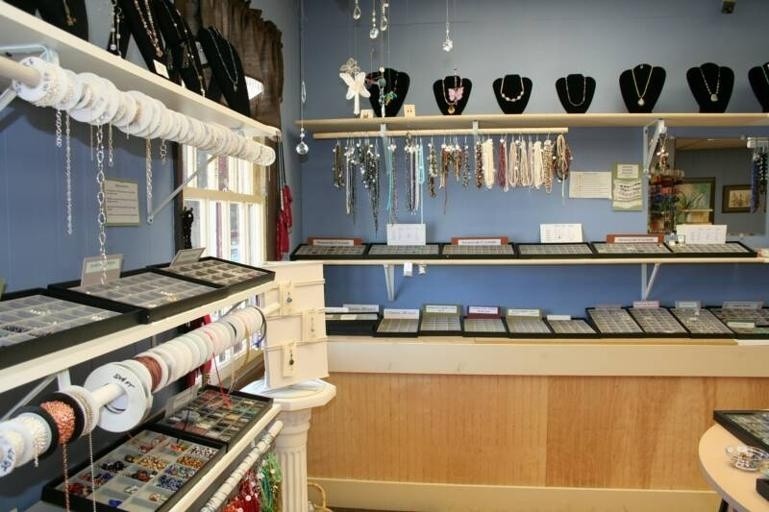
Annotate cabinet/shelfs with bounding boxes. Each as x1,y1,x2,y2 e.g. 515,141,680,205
290,113,769,511
1,2,286,512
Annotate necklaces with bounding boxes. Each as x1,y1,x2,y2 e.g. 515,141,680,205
62,1,240,97
760,64,769,84
698,66,721,102
376,72,399,107
330,133,571,236
441,79,463,115
499,76,525,103
565,76,587,108
631,65,654,107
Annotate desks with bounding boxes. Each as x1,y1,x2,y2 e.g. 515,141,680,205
696,421,769,511
